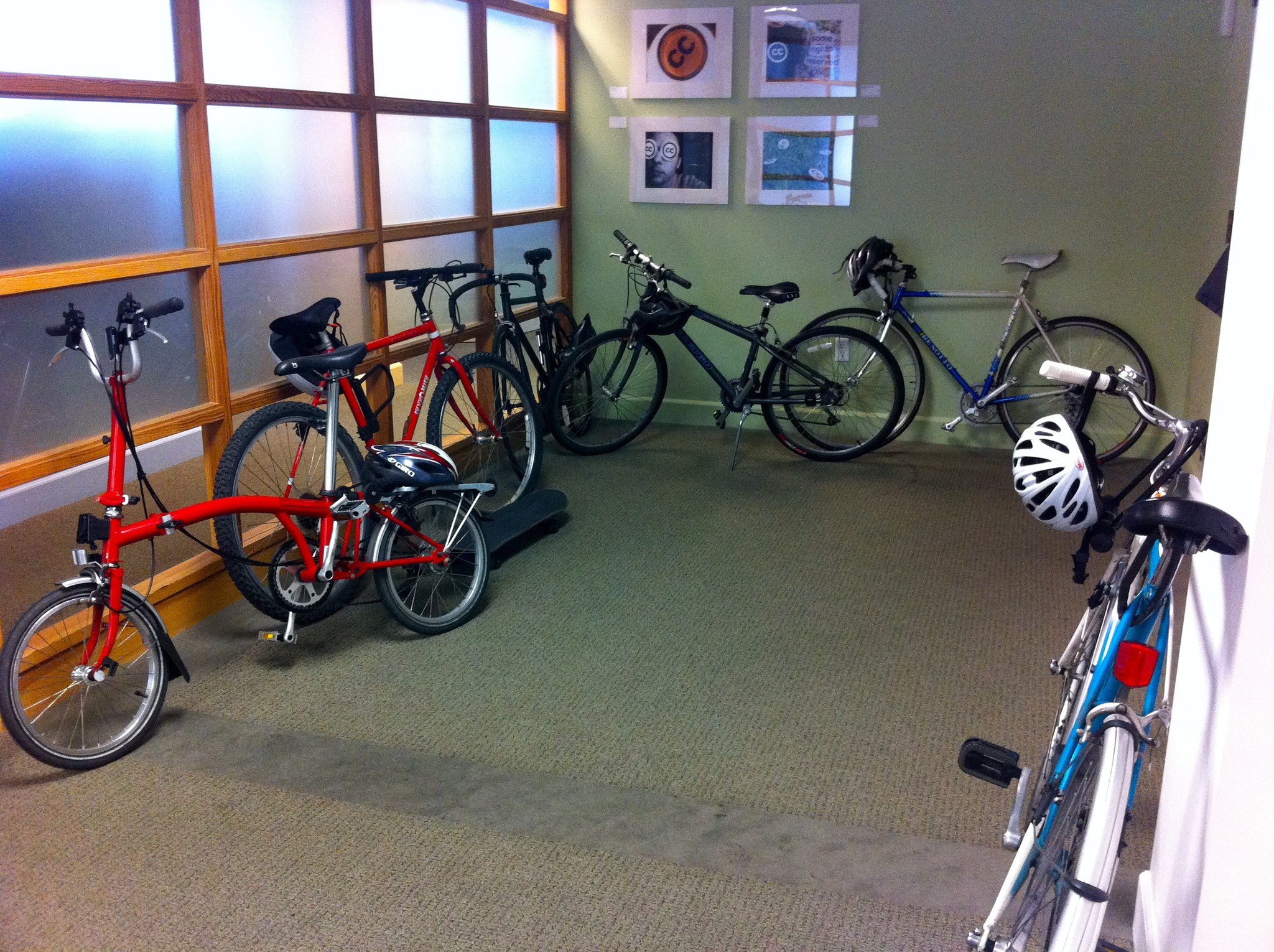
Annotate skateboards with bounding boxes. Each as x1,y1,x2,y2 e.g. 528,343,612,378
439,487,568,570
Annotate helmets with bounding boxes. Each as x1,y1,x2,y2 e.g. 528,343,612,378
846,235,894,296
633,304,699,336
268,327,355,399
1013,412,1105,533
571,312,598,381
370,439,460,489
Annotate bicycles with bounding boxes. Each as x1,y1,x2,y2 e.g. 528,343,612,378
959,360,1248,949
0,248,669,772
550,229,1157,468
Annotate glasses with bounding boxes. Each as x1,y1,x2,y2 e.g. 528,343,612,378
645,139,679,161
